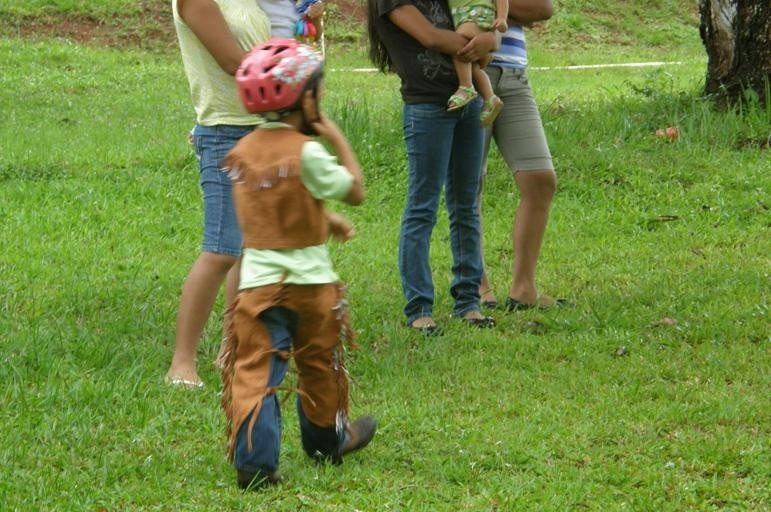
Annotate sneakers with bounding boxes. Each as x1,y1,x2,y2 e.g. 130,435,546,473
236,469,284,490
327,417,376,466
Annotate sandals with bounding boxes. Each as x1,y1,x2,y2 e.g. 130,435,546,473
479,95,504,127
445,86,479,111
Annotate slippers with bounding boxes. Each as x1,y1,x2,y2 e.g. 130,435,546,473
165,373,203,393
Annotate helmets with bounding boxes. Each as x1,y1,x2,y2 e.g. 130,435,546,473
236,40,326,114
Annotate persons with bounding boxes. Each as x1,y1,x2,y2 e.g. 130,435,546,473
470,1,572,311
221,39,378,489
261,1,299,42
296,2,325,52
447,0,509,127
164,0,272,391
369,0,500,334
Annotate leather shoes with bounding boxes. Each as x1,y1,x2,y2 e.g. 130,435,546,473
412,297,568,338
